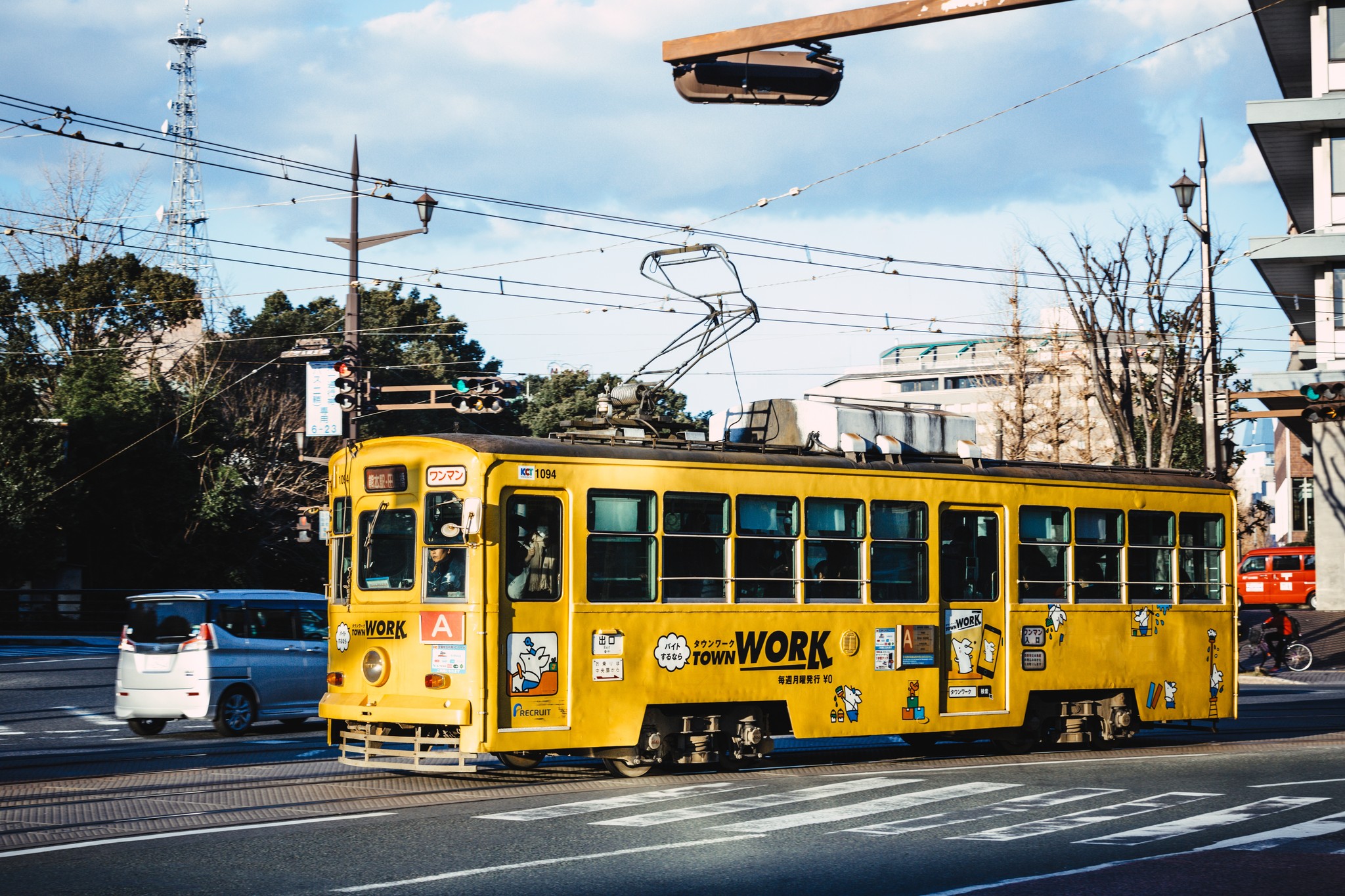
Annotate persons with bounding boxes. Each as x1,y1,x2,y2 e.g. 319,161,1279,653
602,558,648,600
1018,563,1118,599
806,560,851,598
736,540,789,598
1260,605,1293,671
427,546,465,597
508,510,560,598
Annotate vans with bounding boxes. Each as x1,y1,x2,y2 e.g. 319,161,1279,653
1237,545,1317,611
113,591,329,738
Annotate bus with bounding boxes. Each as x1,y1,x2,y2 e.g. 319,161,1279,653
316,245,1245,777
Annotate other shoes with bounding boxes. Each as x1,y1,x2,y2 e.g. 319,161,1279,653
1268,668,1282,673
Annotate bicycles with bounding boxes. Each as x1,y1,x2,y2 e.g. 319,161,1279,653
1238,627,1313,674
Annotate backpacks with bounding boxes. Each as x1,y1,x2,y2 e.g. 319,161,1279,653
1288,615,1302,641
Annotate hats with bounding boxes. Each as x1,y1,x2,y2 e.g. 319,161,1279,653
426,536,446,549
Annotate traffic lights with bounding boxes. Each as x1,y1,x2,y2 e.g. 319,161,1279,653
1299,381,1345,403
451,376,505,394
450,396,505,413
1301,402,1345,423
333,359,357,413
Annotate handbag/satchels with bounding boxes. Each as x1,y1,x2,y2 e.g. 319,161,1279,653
509,543,532,601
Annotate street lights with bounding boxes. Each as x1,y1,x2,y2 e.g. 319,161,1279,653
292,132,440,544
1167,116,1238,480
